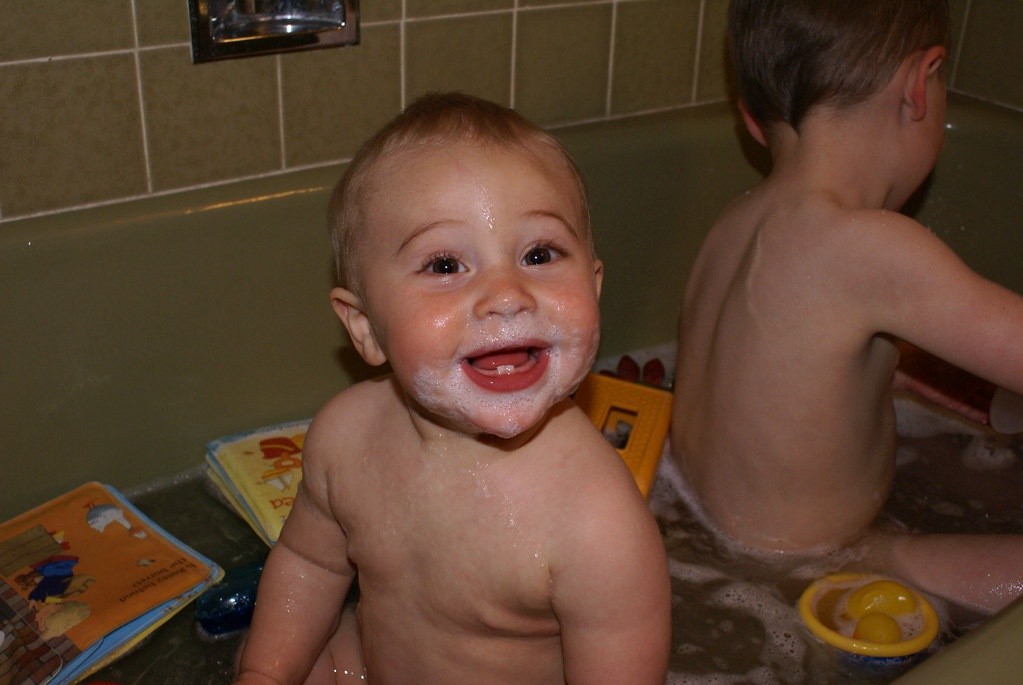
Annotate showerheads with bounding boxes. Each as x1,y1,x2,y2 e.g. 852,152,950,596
208,3,351,44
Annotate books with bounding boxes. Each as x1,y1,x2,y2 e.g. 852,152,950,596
204,416,314,549
0,482,226,685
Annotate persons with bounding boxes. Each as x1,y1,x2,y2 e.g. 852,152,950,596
671,0,1023,617
234,87,673,685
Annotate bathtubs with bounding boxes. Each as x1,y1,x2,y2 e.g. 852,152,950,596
0,92,1023,683
